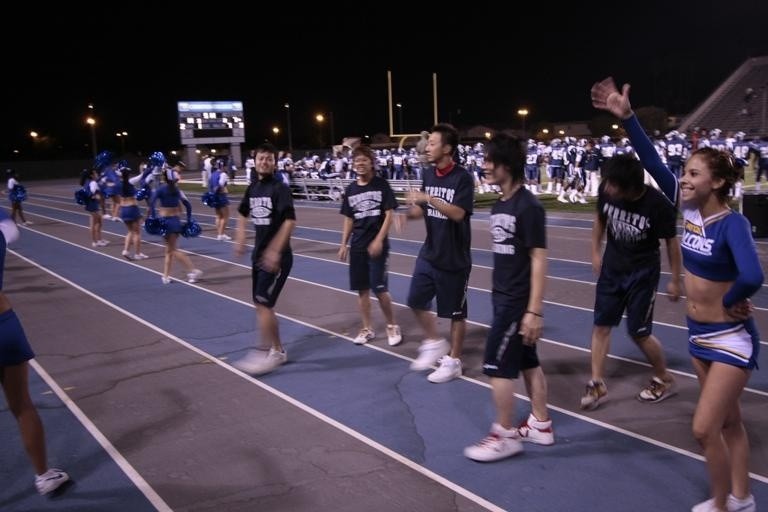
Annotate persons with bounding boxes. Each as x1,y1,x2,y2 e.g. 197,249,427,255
578,75,768,512
399,122,475,383
335,147,404,346
233,142,297,377
1,210,72,497
6,169,32,228
79,152,234,285
250,127,767,202
463,132,557,463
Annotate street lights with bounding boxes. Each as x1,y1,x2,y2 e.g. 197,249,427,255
116,131,130,157
316,112,325,148
284,101,295,153
519,108,528,141
87,102,101,162
395,101,405,134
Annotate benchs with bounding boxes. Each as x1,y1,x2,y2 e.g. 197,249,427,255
285,177,427,208
682,59,768,144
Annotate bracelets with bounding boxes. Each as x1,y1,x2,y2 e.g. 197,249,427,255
524,311,543,318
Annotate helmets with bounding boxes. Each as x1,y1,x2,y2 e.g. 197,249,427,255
304,127,762,164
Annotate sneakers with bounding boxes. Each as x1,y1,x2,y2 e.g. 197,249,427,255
23,221,34,226
353,327,376,345
384,323,402,346
517,412,554,446
90,214,149,260
426,354,463,383
218,234,231,241
35,469,71,496
463,421,524,462
263,347,287,367
580,378,611,411
691,492,756,511
410,337,450,372
187,270,202,284
636,374,679,403
478,182,589,206
162,275,172,285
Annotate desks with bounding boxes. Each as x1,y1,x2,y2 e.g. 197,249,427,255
739,186,768,240
